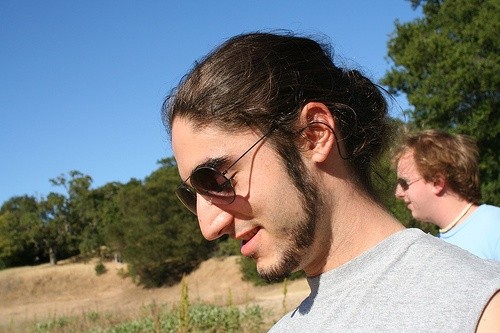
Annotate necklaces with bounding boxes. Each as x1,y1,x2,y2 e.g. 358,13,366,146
439,202,475,234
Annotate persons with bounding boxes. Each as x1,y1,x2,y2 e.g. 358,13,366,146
160,28,500,332
391,125,500,274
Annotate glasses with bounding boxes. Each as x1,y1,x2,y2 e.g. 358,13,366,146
397,177,425,192
175,122,282,216
